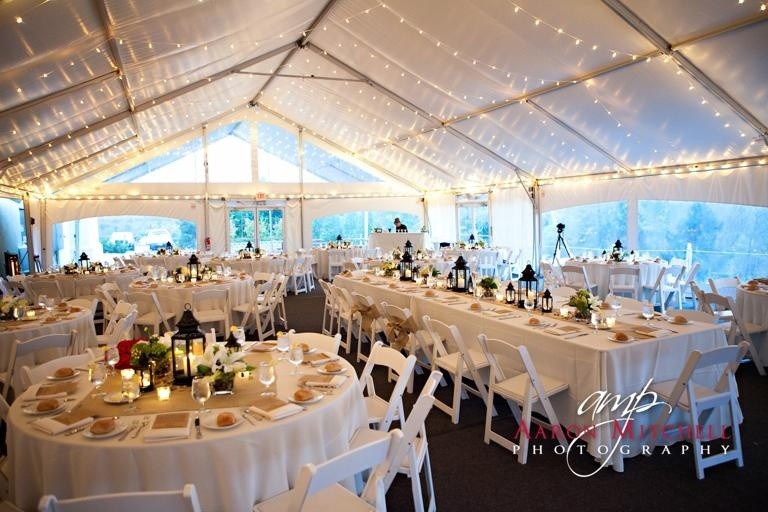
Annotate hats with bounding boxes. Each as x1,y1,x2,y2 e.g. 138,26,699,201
394,218,400,223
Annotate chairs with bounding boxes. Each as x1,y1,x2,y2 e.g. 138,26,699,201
708,279,729,311
96,309,138,346
255,273,275,296
113,255,139,268
233,278,277,341
96,299,137,346
609,267,642,302
211,256,229,273
125,291,175,340
172,302,206,385
330,285,355,355
642,341,750,481
328,250,345,282
698,289,766,375
421,315,498,425
256,273,287,332
357,341,418,432
383,370,444,507
0,330,77,426
290,330,342,356
476,333,571,465
38,483,198,511
381,302,446,393
317,278,339,336
297,255,316,292
539,260,562,288
253,428,405,511
648,265,687,313
675,263,701,310
690,281,702,311
713,276,741,290
353,247,379,270
192,288,231,341
480,248,524,280
351,291,389,364
18,347,97,388
288,256,308,296
560,265,598,296
0,264,141,298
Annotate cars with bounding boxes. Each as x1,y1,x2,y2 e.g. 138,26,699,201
108,231,136,252
146,229,172,250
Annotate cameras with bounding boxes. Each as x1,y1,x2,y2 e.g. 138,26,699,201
557,223,565,234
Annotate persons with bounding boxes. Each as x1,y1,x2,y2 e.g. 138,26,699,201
393,218,408,232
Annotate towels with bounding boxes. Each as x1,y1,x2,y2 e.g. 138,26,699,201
251,340,278,352
142,410,193,443
393,285,421,292
433,295,465,303
309,352,340,367
370,278,389,285
247,398,305,422
349,276,363,280
481,308,517,318
304,373,347,388
634,325,672,338
21,382,79,401
28,409,95,436
544,325,582,336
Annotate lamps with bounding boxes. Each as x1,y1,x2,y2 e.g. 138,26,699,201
542,288,553,313
336,234,343,249
468,234,476,248
446,272,453,289
400,251,412,280
245,241,253,257
135,352,153,393
505,282,515,303
452,256,470,292
165,242,173,255
78,252,89,273
412,266,419,282
518,264,538,309
404,240,413,256
612,240,623,261
187,254,201,282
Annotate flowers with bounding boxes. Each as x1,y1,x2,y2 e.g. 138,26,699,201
392,247,401,261
381,258,397,270
130,336,167,369
569,289,602,312
478,278,498,291
197,342,254,378
421,267,440,277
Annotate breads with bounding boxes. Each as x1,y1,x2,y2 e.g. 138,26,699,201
36,397,59,409
216,412,236,426
748,284,758,290
671,314,687,324
292,389,314,400
54,368,73,378
388,283,396,287
69,306,80,312
470,302,481,310
43,317,56,321
325,361,341,372
49,274,54,279
614,331,629,340
57,301,66,307
529,317,540,324
89,417,116,434
238,275,245,279
32,274,39,277
150,283,155,287
240,271,245,274
599,301,608,309
747,279,756,284
184,282,192,286
298,343,309,351
425,291,433,296
135,281,143,285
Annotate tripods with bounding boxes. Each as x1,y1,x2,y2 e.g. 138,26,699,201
552,234,571,264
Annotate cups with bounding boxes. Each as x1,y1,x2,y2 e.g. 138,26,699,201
26,310,35,320
39,295,48,313
14,306,26,321
259,361,276,399
641,303,654,327
106,347,120,375
606,309,615,329
496,292,504,303
179,274,185,288
610,296,623,318
524,299,535,318
223,269,230,280
559,306,568,317
120,373,141,411
191,377,211,414
427,278,434,289
161,274,167,287
88,362,107,398
202,415,245,431
152,271,158,284
191,278,196,287
277,333,290,362
167,276,175,289
157,387,171,401
233,326,245,343
216,265,223,279
590,309,602,335
288,347,304,377
46,298,54,316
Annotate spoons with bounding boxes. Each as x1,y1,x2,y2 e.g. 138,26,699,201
242,414,255,425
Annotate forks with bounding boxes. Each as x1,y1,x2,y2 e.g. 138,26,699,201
117,416,140,441
244,409,263,421
130,415,152,440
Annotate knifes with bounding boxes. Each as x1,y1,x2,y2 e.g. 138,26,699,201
194,416,203,440
565,333,590,339
635,330,657,338
50,406,70,416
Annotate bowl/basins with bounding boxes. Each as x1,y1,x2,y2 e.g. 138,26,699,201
473,287,483,303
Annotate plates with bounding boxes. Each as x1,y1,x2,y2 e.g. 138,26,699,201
607,335,635,343
301,347,318,354
40,318,62,324
106,393,140,403
21,401,67,415
286,393,324,405
81,424,128,439
667,318,694,325
590,301,602,310
316,365,349,375
46,370,80,380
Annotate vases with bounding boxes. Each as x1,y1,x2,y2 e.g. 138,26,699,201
384,268,393,277
575,307,591,321
481,290,495,300
422,277,427,285
212,372,235,396
